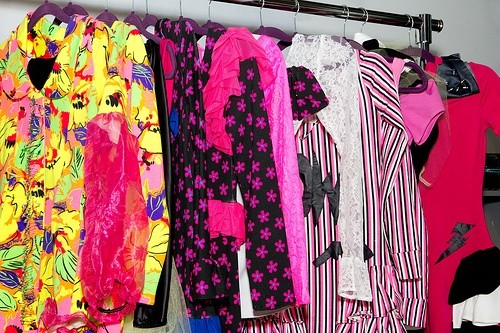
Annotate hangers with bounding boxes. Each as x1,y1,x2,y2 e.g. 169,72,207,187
27,0,429,54
